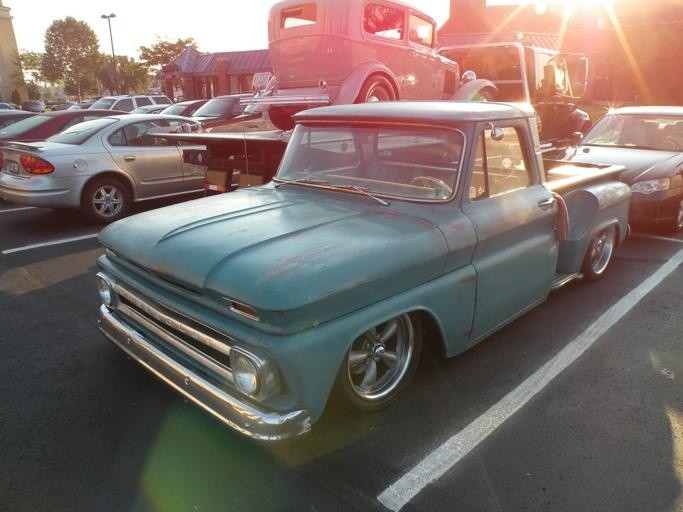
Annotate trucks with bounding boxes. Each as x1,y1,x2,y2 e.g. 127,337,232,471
145,42,593,193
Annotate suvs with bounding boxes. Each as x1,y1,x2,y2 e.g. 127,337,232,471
243,0,499,133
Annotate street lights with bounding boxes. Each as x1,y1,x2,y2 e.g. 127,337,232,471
100,14,122,94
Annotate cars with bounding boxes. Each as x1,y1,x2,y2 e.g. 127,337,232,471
563,106,682,234
1,89,257,226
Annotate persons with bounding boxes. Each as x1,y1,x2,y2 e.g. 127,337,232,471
458,70,487,103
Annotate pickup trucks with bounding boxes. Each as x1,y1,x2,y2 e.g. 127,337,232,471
94,100,633,449
